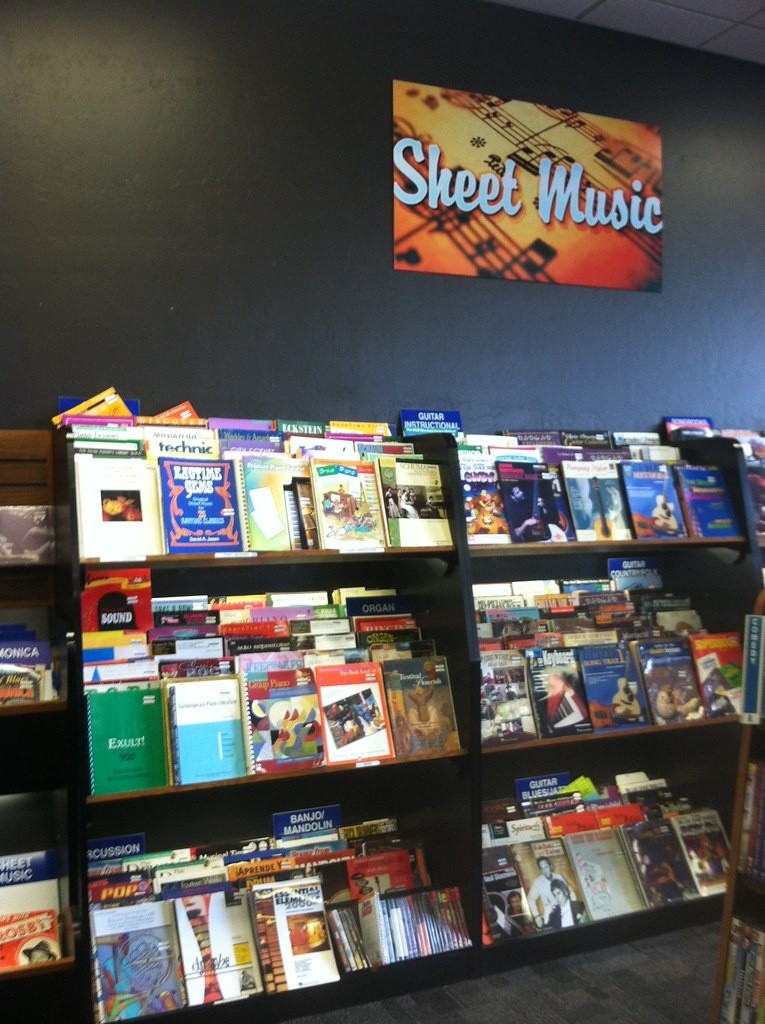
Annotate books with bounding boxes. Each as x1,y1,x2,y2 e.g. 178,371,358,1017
456,416,765,545
81,567,461,796
718,910,765,1024
736,759,765,886
86,804,472,1024
472,554,765,746
480,771,731,946
50,386,464,559
0,504,56,566
0,844,86,976
0,623,68,707
